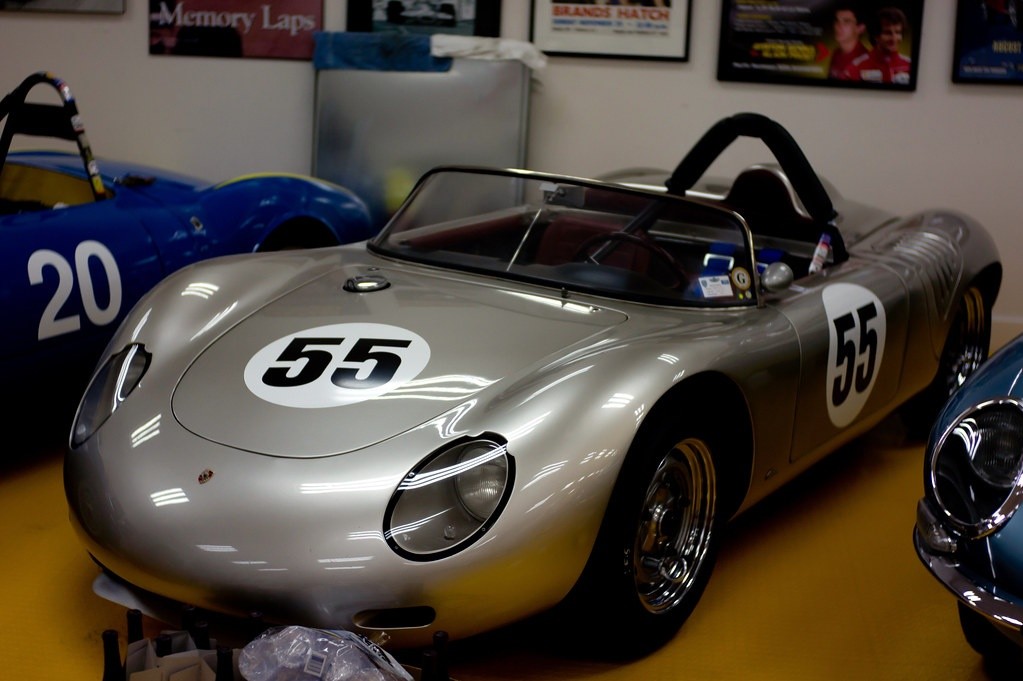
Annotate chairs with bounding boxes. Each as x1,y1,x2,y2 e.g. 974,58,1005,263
540,218,654,285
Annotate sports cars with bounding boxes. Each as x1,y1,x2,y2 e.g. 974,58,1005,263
63,109,1007,670
1,69,374,461
910,332,1023,681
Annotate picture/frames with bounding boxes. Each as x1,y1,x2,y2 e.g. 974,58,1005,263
527,1,693,64
950,1,1023,85
1,1,126,15
148,1,324,62
346,1,503,38
715,0,924,93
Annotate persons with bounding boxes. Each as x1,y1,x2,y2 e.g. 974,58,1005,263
848,8,910,85
830,6,868,78
